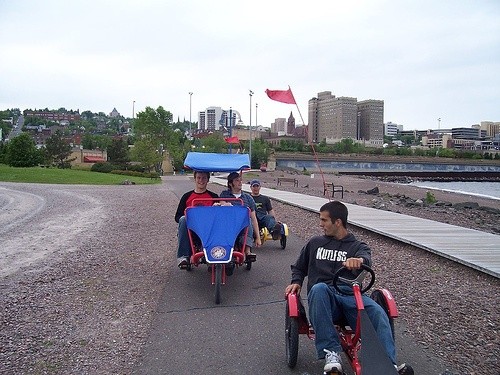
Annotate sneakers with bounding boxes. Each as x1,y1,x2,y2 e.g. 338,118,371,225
324,352,342,373
246,253,256,262
394,363,415,375
177,255,189,266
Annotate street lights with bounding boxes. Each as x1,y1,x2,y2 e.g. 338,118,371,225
189,92,193,130
249,90,254,167
133,101,135,121
256,103,258,137
437,118,441,150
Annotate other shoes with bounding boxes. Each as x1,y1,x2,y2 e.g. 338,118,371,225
272,223,281,240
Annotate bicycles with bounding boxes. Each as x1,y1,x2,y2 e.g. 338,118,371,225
260,221,288,249
285,264,399,375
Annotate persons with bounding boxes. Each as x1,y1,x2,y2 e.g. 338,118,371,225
218,172,261,267
175,170,220,266
284,201,414,375
249,180,282,241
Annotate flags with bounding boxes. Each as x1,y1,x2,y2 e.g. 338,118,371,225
225,136,240,143
264,88,296,105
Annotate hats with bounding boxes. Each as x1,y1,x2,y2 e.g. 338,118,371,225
251,180,260,186
228,172,241,188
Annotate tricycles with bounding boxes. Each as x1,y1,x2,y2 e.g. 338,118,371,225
179,152,256,305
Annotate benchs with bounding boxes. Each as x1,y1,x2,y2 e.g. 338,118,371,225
278,177,299,187
323,182,344,200
